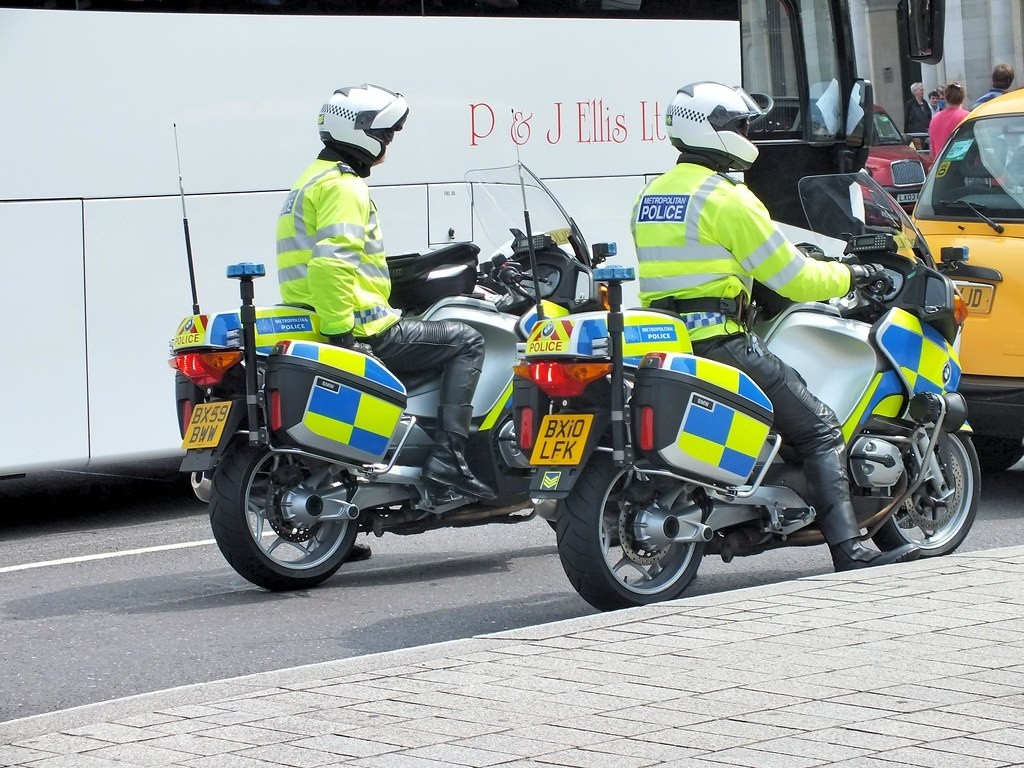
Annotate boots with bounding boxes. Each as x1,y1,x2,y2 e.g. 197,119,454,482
420,404,497,501
814,500,922,572
344,544,371,562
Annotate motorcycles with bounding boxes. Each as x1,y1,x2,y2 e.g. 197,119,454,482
514,106,982,612
167,123,617,591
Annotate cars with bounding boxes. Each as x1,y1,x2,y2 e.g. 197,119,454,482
860,89,1024,472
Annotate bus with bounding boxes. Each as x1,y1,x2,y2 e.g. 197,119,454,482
0,0,945,503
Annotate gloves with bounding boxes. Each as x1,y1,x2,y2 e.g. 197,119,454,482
852,263,892,296
347,341,387,367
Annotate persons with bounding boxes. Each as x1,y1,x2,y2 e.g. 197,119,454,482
904,63,1015,178
631,80,920,571
275,85,505,503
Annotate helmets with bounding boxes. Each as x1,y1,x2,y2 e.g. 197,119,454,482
319,84,409,160
666,82,759,170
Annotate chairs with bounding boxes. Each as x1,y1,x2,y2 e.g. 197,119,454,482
948,136,1009,198
